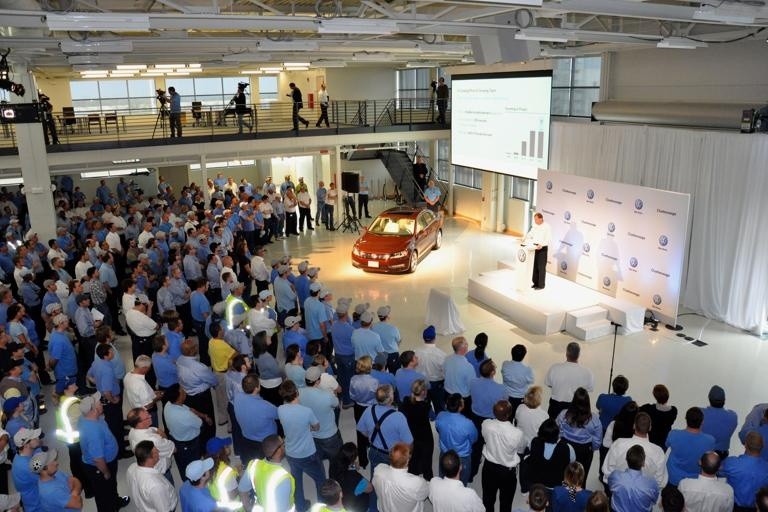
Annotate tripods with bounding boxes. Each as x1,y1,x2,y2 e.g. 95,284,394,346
152,106,178,139
425,90,434,124
343,194,362,235
214,92,237,126
337,193,359,233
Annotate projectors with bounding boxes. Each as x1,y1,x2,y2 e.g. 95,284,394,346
0,46,25,98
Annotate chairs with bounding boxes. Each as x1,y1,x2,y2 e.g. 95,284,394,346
88,114,102,134
192,102,206,127
56,107,76,134
105,110,119,133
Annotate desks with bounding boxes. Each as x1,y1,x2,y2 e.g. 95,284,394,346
58,115,127,133
180,108,224,126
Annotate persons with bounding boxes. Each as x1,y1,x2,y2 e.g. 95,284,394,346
55,377,88,482
525,485,550,512
481,401,527,511
39,95,58,147
413,154,428,202
280,175,297,200
295,178,310,197
306,267,321,286
250,245,270,298
0,330,10,378
608,446,659,512
7,303,36,366
224,314,251,355
235,83,253,136
200,302,228,368
123,355,164,424
200,438,238,512
167,87,182,140
360,176,371,219
639,385,678,446
190,278,213,338
666,408,716,484
283,345,305,389
0,427,9,512
281,255,292,266
283,188,299,236
0,172,283,252
306,334,333,363
286,83,309,133
179,458,216,512
424,180,441,214
126,442,178,511
226,356,251,453
331,304,355,408
326,183,335,230
547,463,591,512
312,355,340,431
428,451,486,512
435,393,478,488
4,393,31,461
320,288,334,328
5,343,44,439
586,490,610,511
557,389,603,489
700,387,737,461
239,435,296,512
601,413,668,512
234,377,276,458
177,340,218,455
597,376,632,482
501,345,534,420
544,343,595,420
273,265,299,331
0,250,119,321
126,296,157,391
316,180,325,225
316,84,332,130
74,294,96,393
442,337,475,416
351,312,386,371
260,290,277,324
96,324,123,389
413,327,446,413
399,382,433,483
305,284,328,342
299,187,312,231
472,360,508,481
278,380,326,512
307,266,324,292
208,322,238,434
372,443,430,511
679,453,734,512
604,402,639,461
349,356,380,468
126,238,253,295
435,77,448,126
394,351,432,404
253,330,286,406
152,334,180,402
226,284,253,333
166,319,185,361
716,431,764,512
45,312,75,406
156,276,176,323
294,260,312,328
163,382,213,486
515,387,550,442
530,420,577,488
163,309,178,332
357,385,413,512
517,213,548,290
77,391,129,512
246,296,277,345
0,357,37,429
371,306,401,372
30,450,82,512
88,345,132,459
345,191,356,223
306,481,353,512
739,403,764,448
351,303,369,328
299,367,343,461
329,443,373,512
466,333,488,376
282,317,307,356
123,281,137,318
271,261,279,282
11,428,42,512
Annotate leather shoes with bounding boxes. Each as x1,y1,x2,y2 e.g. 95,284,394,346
325,225,329,230
115,330,128,336
124,428,130,435
307,226,314,231
302,499,311,512
218,420,228,425
305,120,310,129
38,400,44,404
39,409,47,414
531,285,535,288
124,440,130,446
321,221,326,224
535,287,544,289
41,446,48,452
249,124,254,133
44,381,56,385
120,496,130,506
293,231,299,235
330,226,335,231
117,450,134,459
39,394,45,398
227,431,232,433
285,231,291,237
39,431,45,439
316,221,320,226
124,420,129,426
299,227,304,232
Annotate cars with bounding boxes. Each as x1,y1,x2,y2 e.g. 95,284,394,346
353,208,444,274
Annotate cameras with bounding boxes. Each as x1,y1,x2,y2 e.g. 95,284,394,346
429,80,437,88
238,81,250,96
154,88,167,105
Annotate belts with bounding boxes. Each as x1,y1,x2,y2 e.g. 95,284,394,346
369,443,389,455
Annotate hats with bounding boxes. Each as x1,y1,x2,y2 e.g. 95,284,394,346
138,253,149,260
336,304,349,313
708,386,725,403
46,303,62,315
355,302,370,315
359,311,374,323
284,314,302,328
271,259,280,267
278,265,290,275
50,312,70,325
309,282,322,291
79,391,101,413
13,428,42,448
19,270,31,277
259,230,265,238
0,283,11,289
156,231,165,238
29,451,57,473
280,256,291,265
2,395,28,413
174,218,182,223
185,458,214,481
423,325,436,339
0,492,21,511
229,281,244,291
187,211,195,216
43,280,54,288
204,209,211,214
304,364,323,383
57,227,67,232
216,200,222,204
375,351,388,364
3,357,24,372
377,306,391,319
337,297,352,308
210,242,221,252
7,342,26,359
307,267,320,277
319,288,332,299
134,293,151,304
298,259,308,272
239,202,248,207
198,234,207,242
258,289,272,299
55,376,77,395
206,436,232,457
215,215,222,219
223,210,231,215
10,219,19,224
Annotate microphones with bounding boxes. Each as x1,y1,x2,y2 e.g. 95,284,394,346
609,321,622,328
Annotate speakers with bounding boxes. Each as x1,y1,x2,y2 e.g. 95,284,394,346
341,172,360,193
